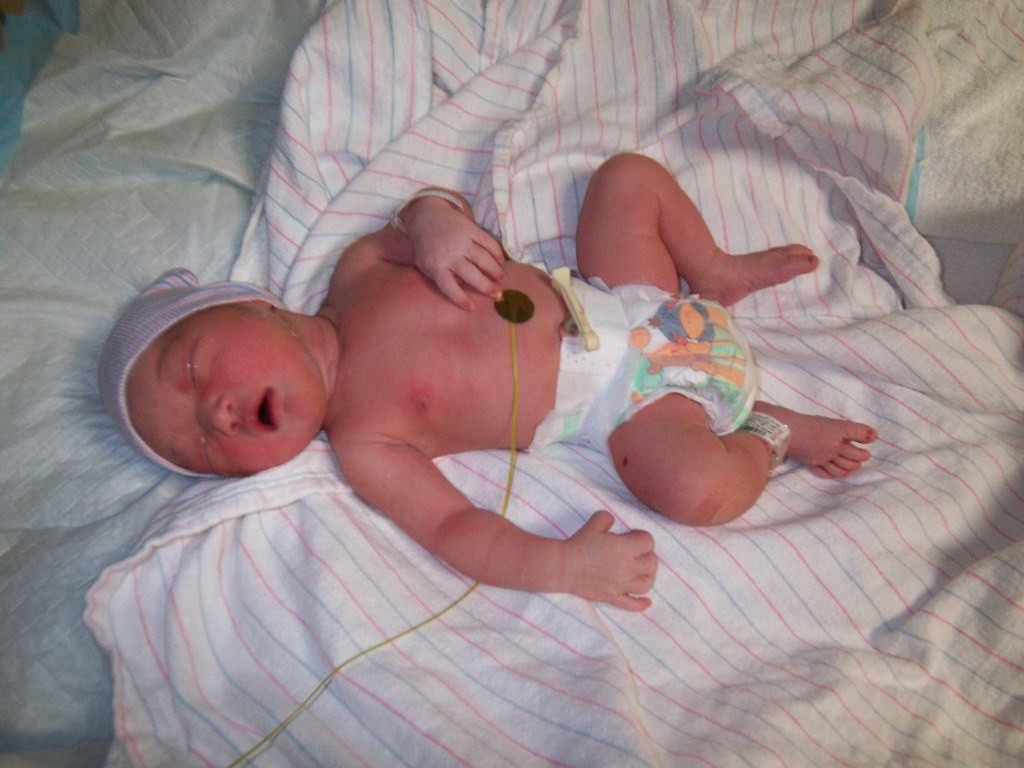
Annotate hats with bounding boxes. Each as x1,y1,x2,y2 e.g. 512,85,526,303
98,269,287,478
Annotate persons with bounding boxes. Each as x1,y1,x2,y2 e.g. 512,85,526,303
98,152,877,614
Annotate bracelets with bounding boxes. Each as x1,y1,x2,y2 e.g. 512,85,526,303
390,191,462,235
